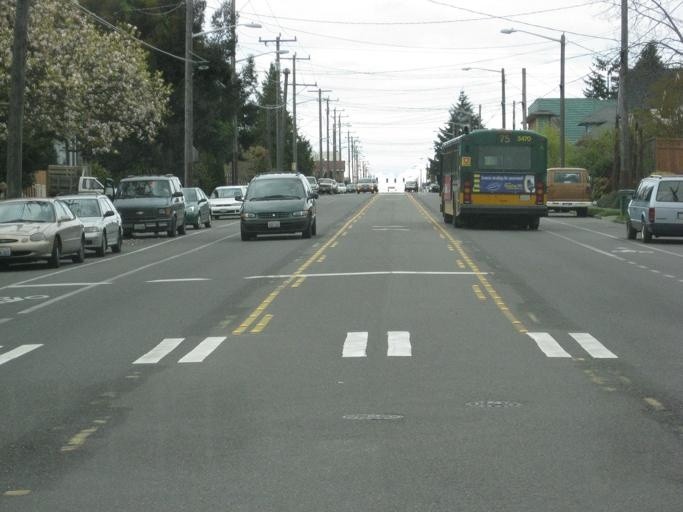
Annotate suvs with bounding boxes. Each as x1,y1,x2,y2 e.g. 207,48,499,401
624,173,682,243
103,173,186,238
235,173,319,241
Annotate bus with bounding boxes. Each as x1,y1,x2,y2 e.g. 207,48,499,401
437,127,551,232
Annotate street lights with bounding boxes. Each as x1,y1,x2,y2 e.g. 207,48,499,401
459,65,506,130
496,27,568,170
181,23,371,186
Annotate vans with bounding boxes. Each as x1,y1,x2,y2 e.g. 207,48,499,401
544,167,593,220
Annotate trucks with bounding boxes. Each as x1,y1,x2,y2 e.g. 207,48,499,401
45,165,106,196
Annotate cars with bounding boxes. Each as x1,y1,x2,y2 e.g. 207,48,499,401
181,187,212,230
208,186,247,219
0,198,86,269
420,181,440,193
45,193,124,258
306,176,379,194
404,181,418,193
